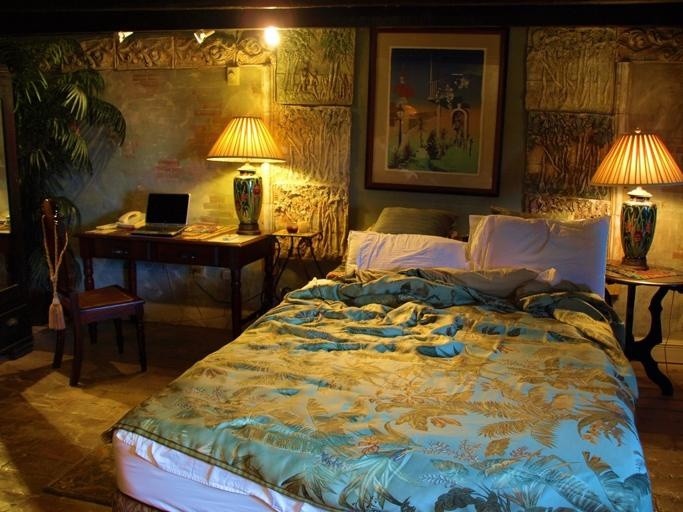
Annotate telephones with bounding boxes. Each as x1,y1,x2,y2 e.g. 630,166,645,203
117,210,146,229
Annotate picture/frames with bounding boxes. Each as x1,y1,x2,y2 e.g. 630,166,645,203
363,24,511,193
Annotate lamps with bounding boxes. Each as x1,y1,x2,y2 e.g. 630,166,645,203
204,114,285,237
588,126,682,273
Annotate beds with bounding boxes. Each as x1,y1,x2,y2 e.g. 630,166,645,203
99,196,656,512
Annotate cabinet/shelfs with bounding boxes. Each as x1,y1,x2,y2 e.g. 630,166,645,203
0,234,39,364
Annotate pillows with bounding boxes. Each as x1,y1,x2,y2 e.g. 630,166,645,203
469,211,611,302
492,205,578,224
372,205,459,241
345,227,470,278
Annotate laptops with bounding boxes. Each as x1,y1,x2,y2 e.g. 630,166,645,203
130,192,191,237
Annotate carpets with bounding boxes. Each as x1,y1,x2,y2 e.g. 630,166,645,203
41,435,119,506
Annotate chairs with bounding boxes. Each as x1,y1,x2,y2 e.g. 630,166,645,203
36,191,148,390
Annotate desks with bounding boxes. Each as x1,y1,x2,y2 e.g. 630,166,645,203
603,260,682,399
260,231,323,309
77,221,276,354
